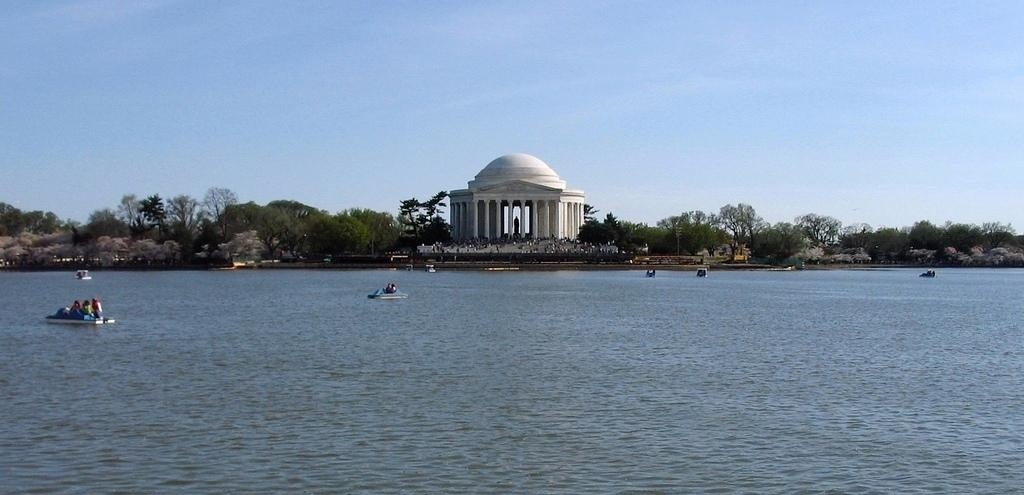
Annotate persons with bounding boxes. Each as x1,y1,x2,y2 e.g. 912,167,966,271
431,234,649,260
70,298,103,318
386,283,396,293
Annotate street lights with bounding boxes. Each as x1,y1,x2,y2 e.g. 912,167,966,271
674,220,682,256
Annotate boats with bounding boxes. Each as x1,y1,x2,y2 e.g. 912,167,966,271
646,269,656,278
45,307,114,328
917,271,936,278
424,264,436,273
695,268,707,278
366,288,410,300
72,270,93,280
405,264,413,272
